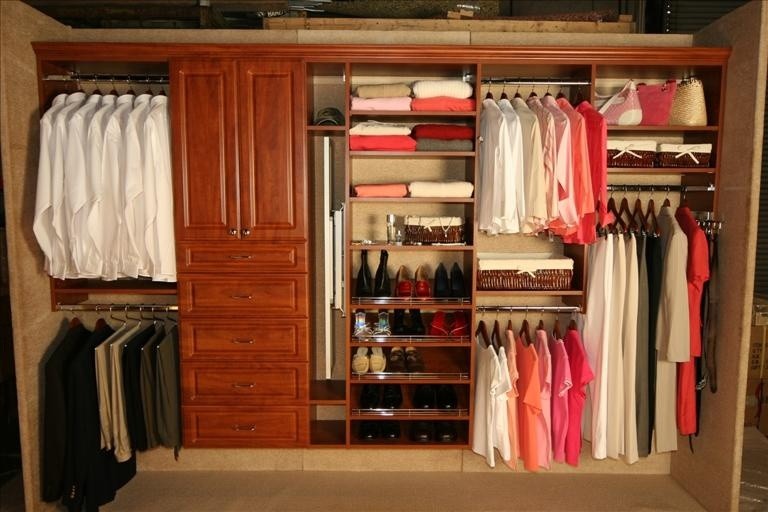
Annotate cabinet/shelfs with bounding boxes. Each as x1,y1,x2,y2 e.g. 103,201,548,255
171,56,309,449
36,55,183,296
307,63,479,450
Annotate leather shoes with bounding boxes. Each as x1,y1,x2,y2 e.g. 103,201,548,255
358,420,457,440
362,384,457,409
355,250,465,299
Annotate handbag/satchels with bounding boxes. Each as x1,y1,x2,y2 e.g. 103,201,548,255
594,78,709,126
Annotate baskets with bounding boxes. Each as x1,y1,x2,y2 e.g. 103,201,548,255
657,143,712,168
607,139,656,168
477,253,575,290
404,214,465,242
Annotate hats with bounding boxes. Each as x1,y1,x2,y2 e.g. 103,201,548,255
315,107,344,126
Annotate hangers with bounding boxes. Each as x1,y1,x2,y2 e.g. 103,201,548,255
476,304,587,361
68,301,179,351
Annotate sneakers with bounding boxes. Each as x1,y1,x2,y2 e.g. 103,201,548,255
352,347,423,374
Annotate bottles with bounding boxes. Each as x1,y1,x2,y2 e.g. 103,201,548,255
387,214,395,245
395,230,402,247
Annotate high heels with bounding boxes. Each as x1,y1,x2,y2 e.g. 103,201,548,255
354,309,467,342
